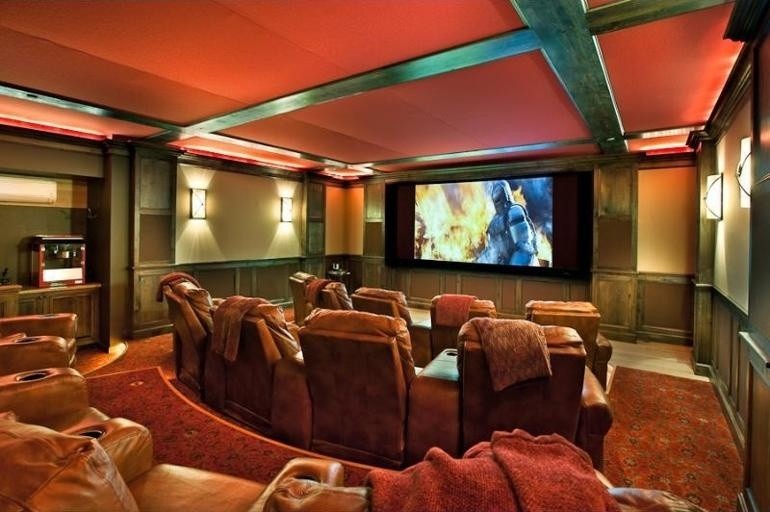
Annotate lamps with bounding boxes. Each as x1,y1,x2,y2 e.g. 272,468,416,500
190,188,207,219
281,197,293,222
735,137,751,208
703,173,723,220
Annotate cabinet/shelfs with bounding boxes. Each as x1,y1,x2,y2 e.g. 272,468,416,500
20,291,101,349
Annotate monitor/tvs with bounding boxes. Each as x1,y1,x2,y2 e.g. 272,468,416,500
384,170,593,280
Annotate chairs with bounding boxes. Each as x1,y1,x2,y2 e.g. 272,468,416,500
2,310,705,511
157,271,614,478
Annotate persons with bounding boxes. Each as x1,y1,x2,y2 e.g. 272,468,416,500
472,179,541,267
